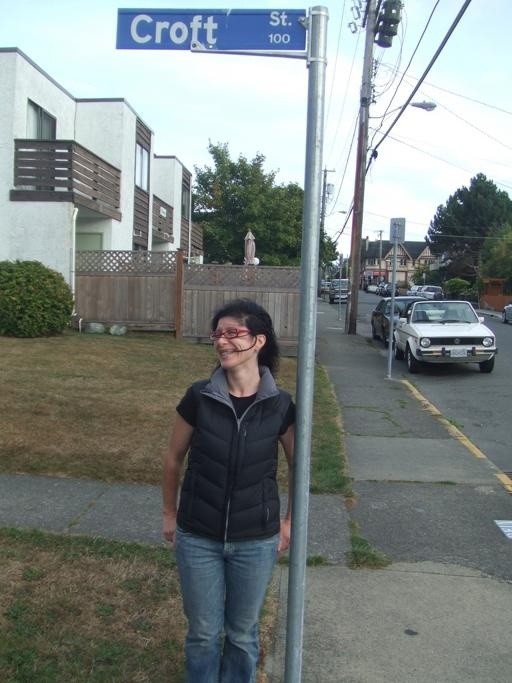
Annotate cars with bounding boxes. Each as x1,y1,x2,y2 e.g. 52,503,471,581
369,297,427,344
500,302,512,324
387,301,498,372
320,279,332,294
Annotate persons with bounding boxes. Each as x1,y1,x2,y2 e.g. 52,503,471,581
160,297,296,683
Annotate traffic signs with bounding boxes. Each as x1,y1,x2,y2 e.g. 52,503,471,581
115,4,310,53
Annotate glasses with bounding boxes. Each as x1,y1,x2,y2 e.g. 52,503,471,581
208,328,251,341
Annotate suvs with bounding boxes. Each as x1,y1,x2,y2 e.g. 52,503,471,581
327,279,352,303
367,281,445,301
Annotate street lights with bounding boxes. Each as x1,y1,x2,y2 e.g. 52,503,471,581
321,210,346,237
342,100,437,335
